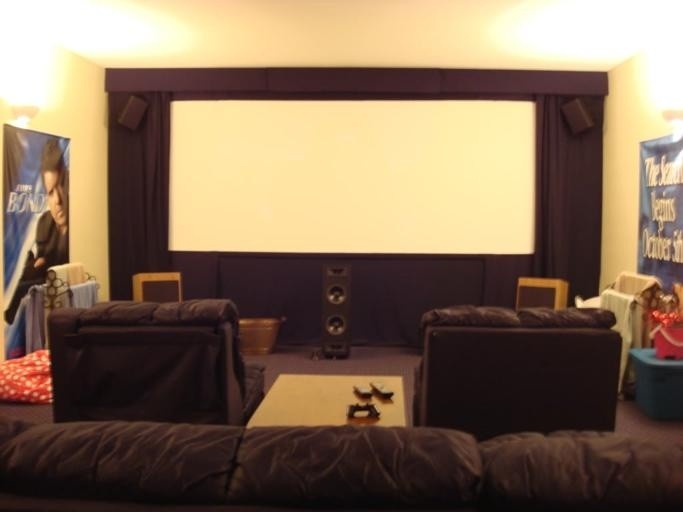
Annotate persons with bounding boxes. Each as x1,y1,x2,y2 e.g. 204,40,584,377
33,139,69,282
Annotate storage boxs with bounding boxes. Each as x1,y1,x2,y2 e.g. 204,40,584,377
629,348,683,420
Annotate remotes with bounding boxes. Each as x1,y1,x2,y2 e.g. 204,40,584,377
371,380,395,399
353,383,373,397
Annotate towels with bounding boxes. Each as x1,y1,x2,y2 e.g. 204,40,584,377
26,260,101,353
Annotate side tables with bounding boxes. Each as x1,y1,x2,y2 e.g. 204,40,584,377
246,374,407,428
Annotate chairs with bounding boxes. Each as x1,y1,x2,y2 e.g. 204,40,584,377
45,300,265,423
412,305,623,443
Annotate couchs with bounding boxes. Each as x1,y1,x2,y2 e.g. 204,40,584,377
0,420,682,512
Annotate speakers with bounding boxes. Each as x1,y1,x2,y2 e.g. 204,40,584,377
321,261,352,359
118,96,150,132
560,99,596,139
513,276,569,313
132,270,182,304
